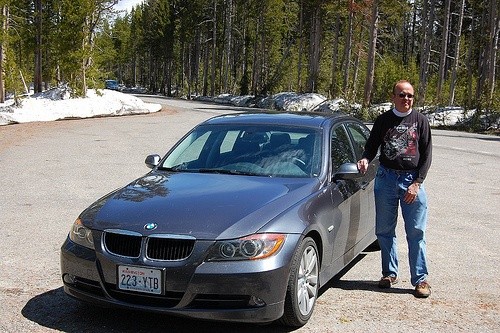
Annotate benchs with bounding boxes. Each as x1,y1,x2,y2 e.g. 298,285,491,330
263,131,316,156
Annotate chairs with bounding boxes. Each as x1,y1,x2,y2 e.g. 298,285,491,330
225,141,260,164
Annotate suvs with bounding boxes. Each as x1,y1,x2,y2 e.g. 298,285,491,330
105,80,119,91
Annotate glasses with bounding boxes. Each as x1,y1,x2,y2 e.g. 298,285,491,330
395,94,415,99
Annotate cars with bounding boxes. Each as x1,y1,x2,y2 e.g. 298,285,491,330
61,111,378,329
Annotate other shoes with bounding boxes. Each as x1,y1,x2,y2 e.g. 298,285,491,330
378,274,400,288
414,278,432,297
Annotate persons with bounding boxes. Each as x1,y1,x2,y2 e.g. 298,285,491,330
356,80,432,297
